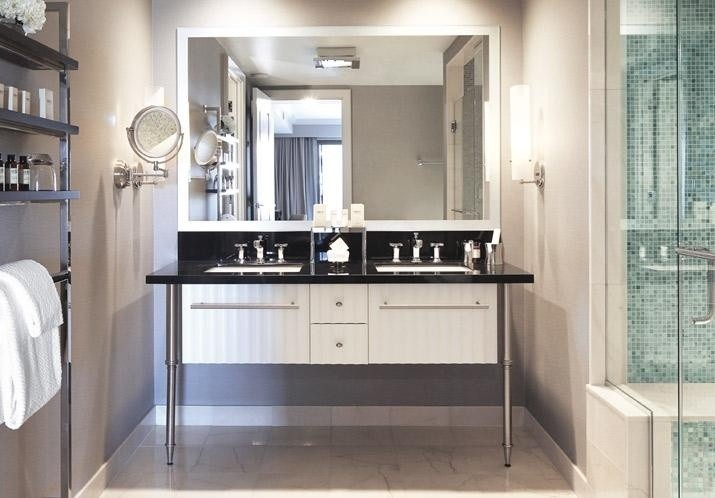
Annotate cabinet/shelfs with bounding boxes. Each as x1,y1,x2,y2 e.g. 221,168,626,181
179,286,310,363
368,287,497,365
0,0,85,494
309,288,366,365
198,102,240,220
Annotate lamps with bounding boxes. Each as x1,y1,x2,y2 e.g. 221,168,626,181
313,45,362,72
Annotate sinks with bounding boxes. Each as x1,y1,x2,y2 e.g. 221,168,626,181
202,260,306,274
371,260,474,274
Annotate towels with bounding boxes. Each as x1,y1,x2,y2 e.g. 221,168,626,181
2,258,66,429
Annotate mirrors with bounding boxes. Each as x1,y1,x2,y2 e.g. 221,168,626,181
115,107,186,193
189,128,227,180
175,22,500,230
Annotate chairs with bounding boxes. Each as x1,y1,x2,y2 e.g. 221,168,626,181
288,212,307,221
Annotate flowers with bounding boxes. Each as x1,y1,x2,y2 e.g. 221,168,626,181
221,113,235,135
0,1,50,37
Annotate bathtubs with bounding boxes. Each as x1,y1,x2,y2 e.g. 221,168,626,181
625,381,715,497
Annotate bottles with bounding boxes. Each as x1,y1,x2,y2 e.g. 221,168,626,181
5,153,19,192
25,153,58,192
17,154,31,191
0,153,6,192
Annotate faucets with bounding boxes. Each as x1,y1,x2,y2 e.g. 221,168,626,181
252,238,266,264
410,232,424,263
